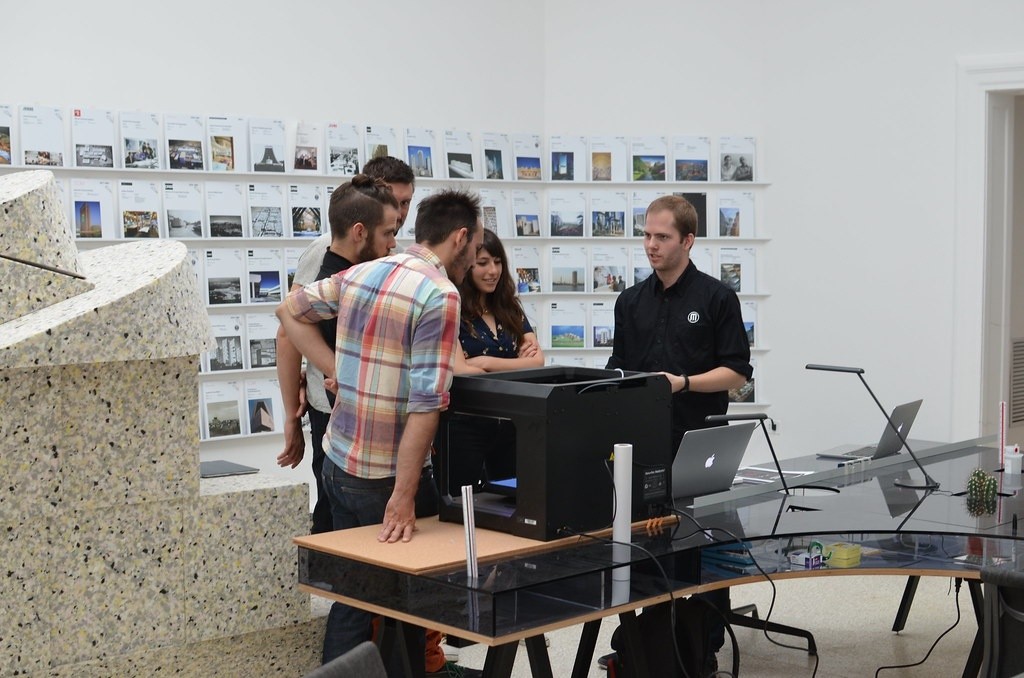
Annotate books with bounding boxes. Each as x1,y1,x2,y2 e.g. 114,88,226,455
0,103,758,440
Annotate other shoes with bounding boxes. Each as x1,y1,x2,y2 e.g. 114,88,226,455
425,662,482,678
598,651,616,669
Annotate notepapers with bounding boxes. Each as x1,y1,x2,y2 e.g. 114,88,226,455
825,541,861,559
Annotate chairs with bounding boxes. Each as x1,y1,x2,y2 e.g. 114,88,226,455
299,640,388,678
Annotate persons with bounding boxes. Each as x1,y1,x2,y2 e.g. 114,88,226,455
451,228,545,375
597,194,755,670
611,276,618,292
276,155,416,469
605,274,611,285
275,192,484,678
732,156,753,181
313,175,483,678
617,275,626,292
721,156,736,180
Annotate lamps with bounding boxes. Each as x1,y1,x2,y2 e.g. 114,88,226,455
806,363,942,489
705,412,840,512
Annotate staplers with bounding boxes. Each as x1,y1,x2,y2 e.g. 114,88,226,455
702,542,755,567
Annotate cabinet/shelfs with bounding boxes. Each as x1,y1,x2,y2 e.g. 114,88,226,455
0,164,774,444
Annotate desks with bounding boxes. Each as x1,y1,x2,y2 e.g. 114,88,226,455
291,438,1024,678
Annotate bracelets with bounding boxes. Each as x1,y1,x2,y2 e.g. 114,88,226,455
679,374,690,394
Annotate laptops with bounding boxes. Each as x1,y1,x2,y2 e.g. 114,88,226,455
816,399,924,459
669,422,756,498
